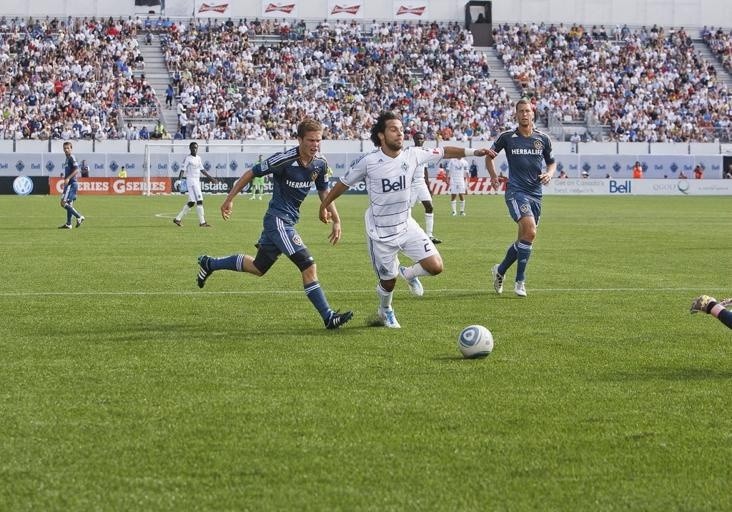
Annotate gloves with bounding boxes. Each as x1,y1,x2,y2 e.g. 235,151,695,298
690,295,717,315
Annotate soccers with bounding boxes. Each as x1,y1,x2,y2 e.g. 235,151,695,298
458,325,494,359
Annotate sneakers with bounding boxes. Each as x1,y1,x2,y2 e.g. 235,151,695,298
450,211,457,217
460,213,466,216
400,266,424,297
195,255,214,288
379,305,401,328
58,224,72,230
173,218,183,227
75,215,85,228
514,280,527,297
200,222,210,227
491,263,505,294
430,237,443,243
324,310,353,329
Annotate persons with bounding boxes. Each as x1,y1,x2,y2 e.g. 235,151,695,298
317,113,499,328
404,131,444,243
484,100,557,297
58,142,85,229
247,155,264,201
173,142,218,227
690,295,732,330
195,117,354,330
445,157,469,217
